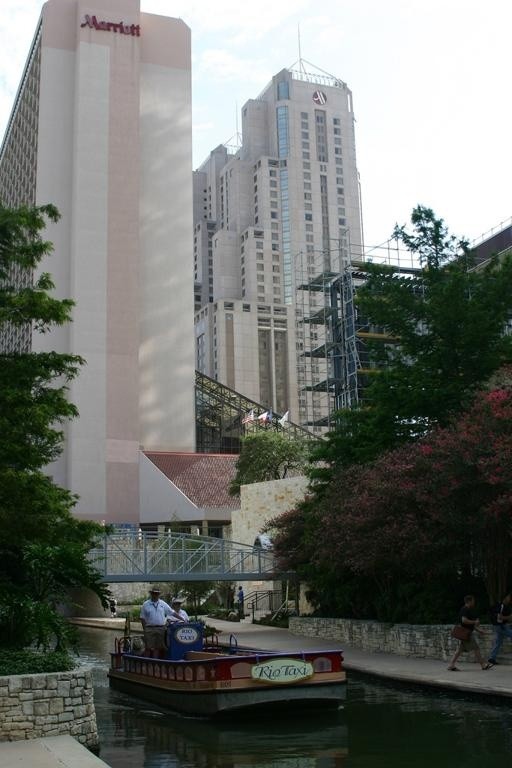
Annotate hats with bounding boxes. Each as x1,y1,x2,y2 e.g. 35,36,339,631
149,587,161,595
173,598,184,603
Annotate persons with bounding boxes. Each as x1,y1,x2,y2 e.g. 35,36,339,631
237,584,244,615
225,584,234,609
447,593,495,671
487,590,512,664
138,585,188,659
166,594,192,623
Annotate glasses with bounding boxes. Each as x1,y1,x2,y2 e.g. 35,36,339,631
174,603,181,605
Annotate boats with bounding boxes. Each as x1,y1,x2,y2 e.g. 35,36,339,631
106,616,350,714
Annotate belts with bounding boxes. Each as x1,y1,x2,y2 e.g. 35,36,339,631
146,625,164,628
